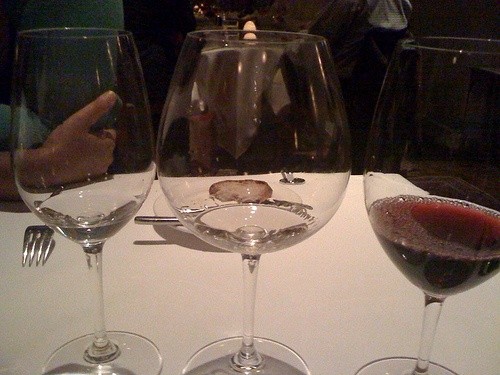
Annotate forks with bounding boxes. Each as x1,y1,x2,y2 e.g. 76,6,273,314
21,186,64,266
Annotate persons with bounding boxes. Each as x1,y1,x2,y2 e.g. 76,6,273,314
120,0,423,175
0,0,125,202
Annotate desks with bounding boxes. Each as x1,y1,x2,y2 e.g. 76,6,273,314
0,172,500,375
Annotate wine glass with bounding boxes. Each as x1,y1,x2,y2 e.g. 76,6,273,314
353,33,500,375
152,29,352,375
10,24,159,375
